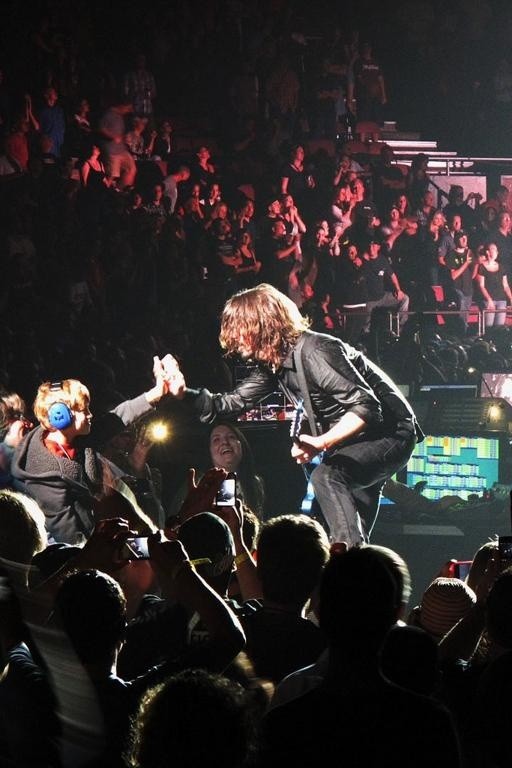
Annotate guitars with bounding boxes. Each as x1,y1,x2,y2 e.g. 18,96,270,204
289,399,330,536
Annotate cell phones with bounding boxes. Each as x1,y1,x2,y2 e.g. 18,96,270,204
498,536,512,570
215,472,237,511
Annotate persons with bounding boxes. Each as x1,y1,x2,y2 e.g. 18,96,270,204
0,354,512,768
1,30,510,398
162,281,425,545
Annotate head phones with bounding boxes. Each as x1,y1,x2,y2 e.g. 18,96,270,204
48,380,73,430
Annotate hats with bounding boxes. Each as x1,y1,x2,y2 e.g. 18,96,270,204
419,576,482,636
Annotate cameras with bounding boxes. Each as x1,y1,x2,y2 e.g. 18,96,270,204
452,561,473,583
118,534,151,560
